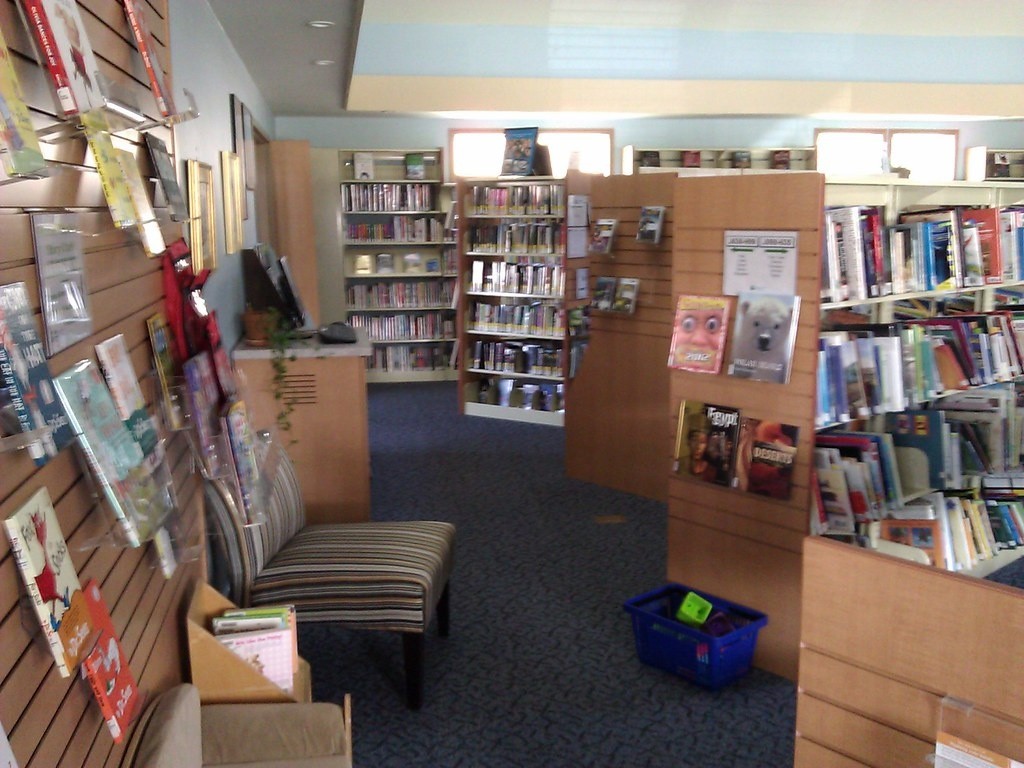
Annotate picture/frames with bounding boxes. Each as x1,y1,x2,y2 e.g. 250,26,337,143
187,158,218,276
222,150,244,254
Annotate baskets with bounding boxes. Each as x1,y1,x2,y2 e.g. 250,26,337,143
624,581,769,689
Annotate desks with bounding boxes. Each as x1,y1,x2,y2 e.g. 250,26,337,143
231,326,373,526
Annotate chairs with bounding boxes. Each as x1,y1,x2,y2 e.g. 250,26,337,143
199,435,459,710
119,682,353,768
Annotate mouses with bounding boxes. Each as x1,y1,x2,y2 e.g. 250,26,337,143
331,321,346,326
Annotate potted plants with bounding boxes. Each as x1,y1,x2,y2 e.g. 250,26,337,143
238,306,334,463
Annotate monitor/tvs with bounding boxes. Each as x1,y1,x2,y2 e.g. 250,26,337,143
278,255,307,327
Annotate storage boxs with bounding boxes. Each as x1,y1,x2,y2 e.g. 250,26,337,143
622,580,767,690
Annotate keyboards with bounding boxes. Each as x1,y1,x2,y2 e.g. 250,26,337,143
318,324,357,344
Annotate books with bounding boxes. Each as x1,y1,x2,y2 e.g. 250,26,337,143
0,0,1024,768
2,487,95,678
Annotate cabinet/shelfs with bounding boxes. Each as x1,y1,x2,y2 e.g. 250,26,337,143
448,176,565,428
339,179,461,384
674,173,1024,683
964,146,1024,181
